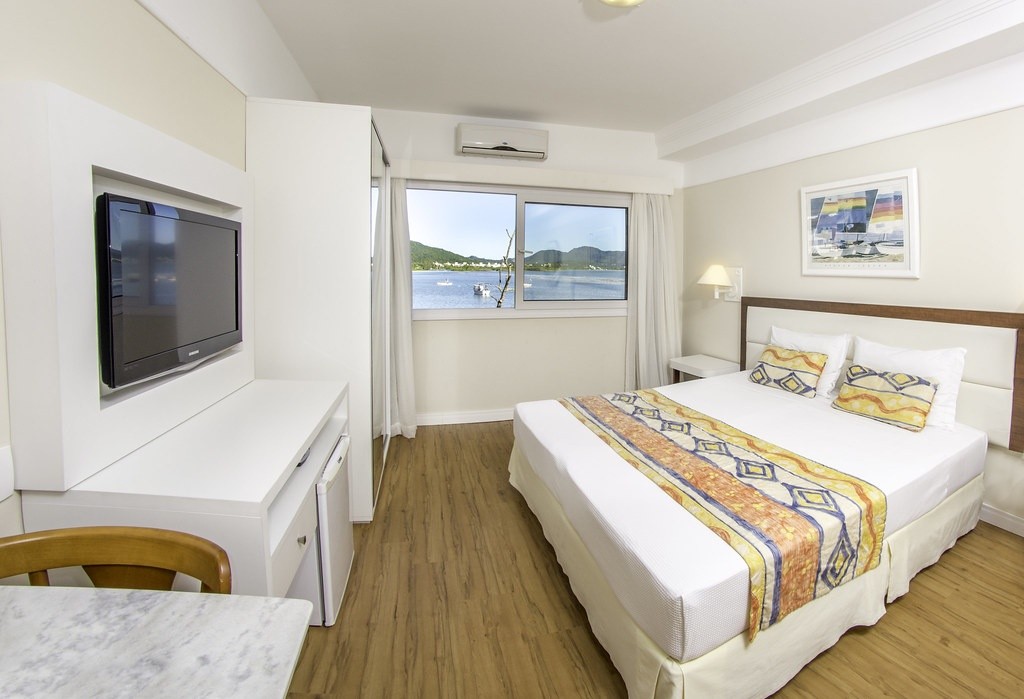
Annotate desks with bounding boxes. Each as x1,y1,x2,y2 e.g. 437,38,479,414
0,585,314,699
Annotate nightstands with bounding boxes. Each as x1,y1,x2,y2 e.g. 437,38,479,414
669,354,740,384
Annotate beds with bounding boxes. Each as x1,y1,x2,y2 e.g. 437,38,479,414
507,297,1024,699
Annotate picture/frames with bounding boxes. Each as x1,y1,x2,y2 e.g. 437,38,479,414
800,168,920,280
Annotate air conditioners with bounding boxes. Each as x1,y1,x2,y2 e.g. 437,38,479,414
456,123,549,160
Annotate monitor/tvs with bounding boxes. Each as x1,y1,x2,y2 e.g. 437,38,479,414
97,193,243,389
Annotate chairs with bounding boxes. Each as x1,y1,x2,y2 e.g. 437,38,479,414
0,525,231,594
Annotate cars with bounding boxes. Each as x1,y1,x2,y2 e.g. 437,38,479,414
153,264,185,292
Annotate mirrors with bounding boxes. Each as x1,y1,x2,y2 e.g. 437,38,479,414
372,120,386,508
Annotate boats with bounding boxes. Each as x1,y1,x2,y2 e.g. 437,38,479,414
473,284,491,296
437,281,453,287
524,283,534,288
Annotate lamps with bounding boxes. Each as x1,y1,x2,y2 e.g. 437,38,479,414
696,264,743,302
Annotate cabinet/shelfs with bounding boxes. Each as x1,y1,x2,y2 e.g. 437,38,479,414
21,377,354,626
245,97,391,525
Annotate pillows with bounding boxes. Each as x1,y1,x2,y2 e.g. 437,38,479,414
770,325,850,399
853,335,968,435
748,345,829,398
830,364,938,432
